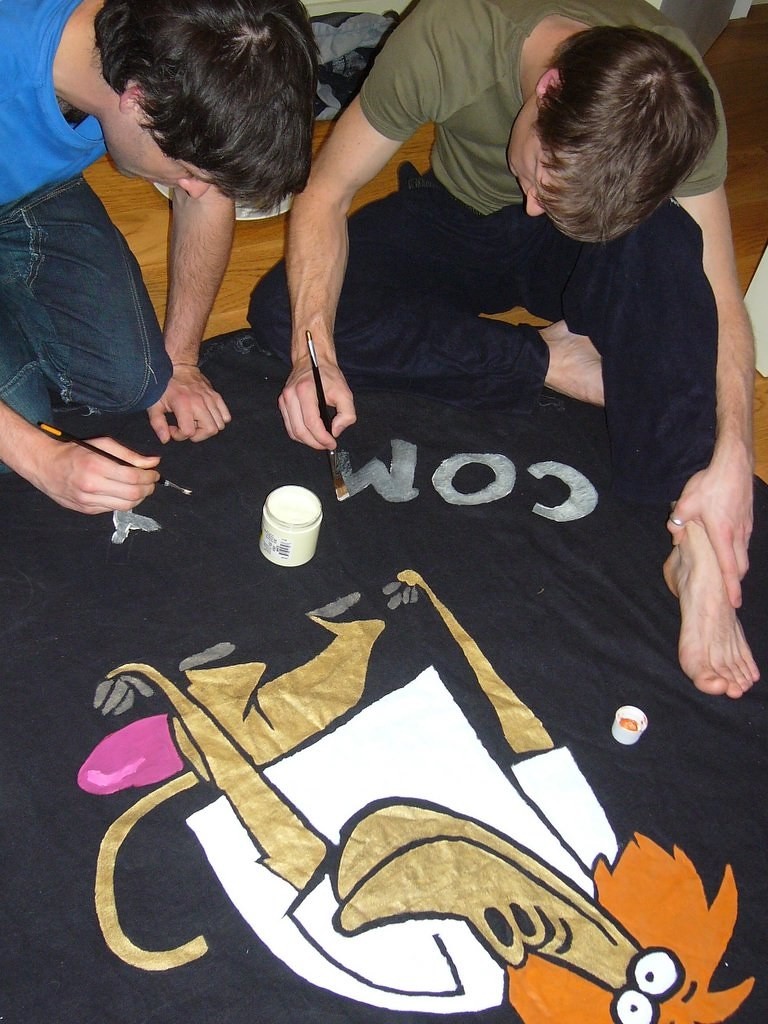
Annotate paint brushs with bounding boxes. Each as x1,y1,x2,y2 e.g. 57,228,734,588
37,420,196,497
305,330,350,500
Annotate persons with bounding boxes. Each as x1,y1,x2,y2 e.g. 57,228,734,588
246,0,762,698
0,0,323,516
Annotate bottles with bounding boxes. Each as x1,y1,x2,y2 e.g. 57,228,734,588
259,486,323,567
